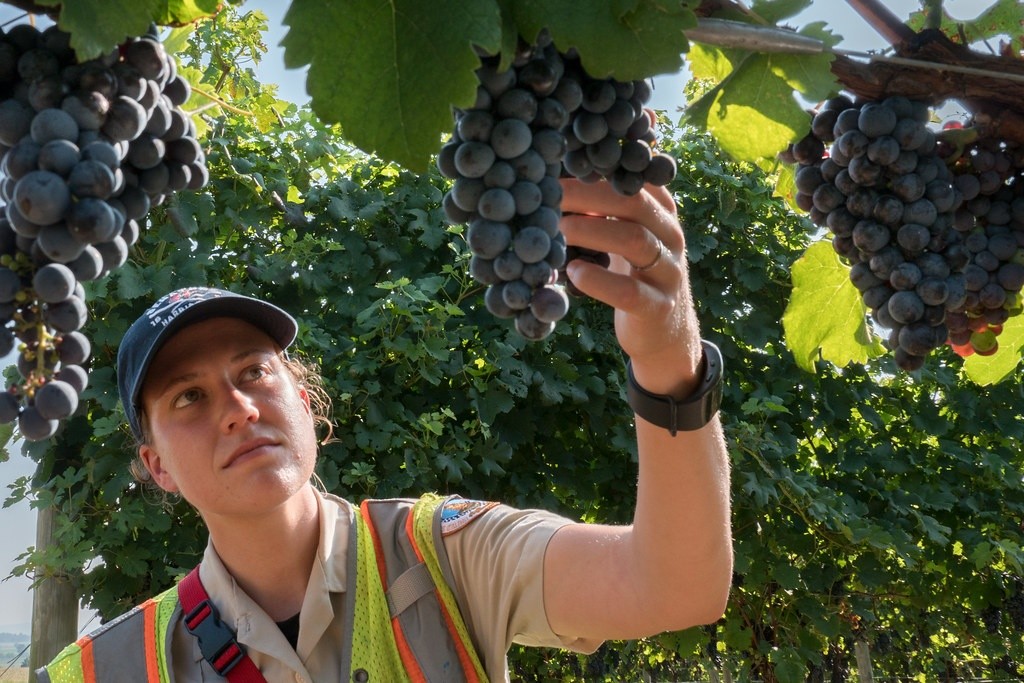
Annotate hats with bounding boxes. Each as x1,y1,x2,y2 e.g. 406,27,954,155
115,285,299,443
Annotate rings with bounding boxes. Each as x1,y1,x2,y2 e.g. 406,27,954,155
630,238,664,271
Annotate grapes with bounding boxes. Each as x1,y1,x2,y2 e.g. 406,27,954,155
0,0,213,440
777,91,1024,372
435,27,676,340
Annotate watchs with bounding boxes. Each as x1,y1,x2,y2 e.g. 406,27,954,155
625,340,722,432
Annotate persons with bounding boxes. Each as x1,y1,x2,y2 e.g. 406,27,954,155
35,111,735,683
